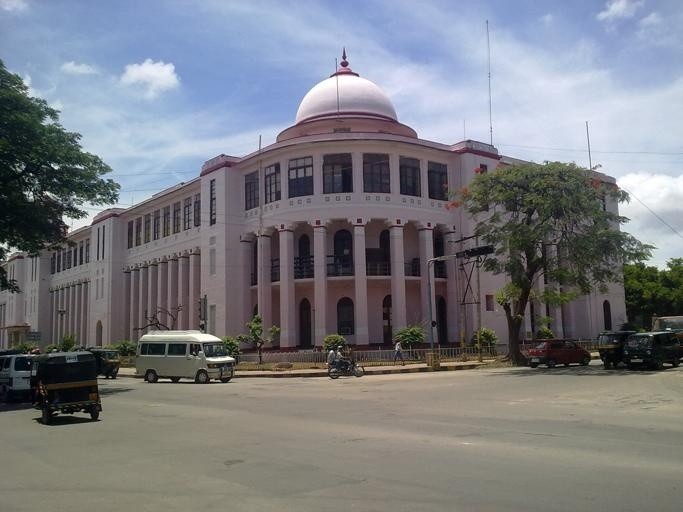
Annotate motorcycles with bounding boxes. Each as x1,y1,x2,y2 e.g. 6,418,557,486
327,357,365,379
30,351,102,423
90,348,120,379
598,331,636,370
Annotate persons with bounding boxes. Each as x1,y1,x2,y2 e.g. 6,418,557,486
335,345,351,373
327,347,343,373
391,340,405,366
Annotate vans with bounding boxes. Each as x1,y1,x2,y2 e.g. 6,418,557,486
1,354,41,402
135,330,236,383
623,331,680,369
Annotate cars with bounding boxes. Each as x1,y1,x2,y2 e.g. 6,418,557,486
527,339,591,369
